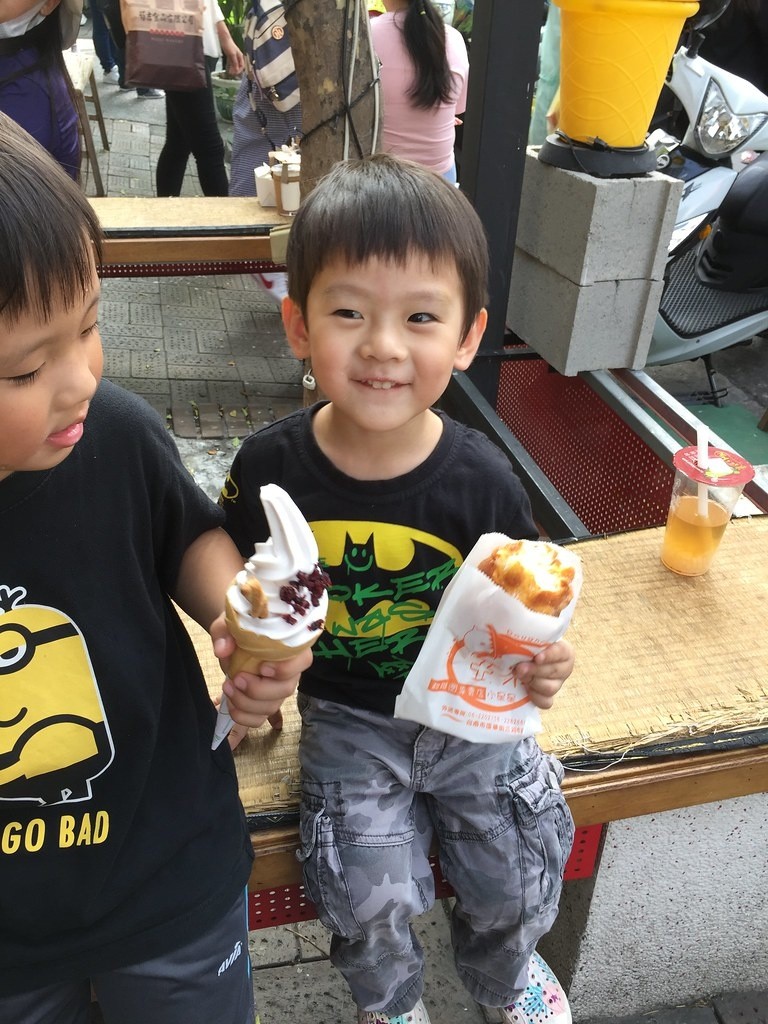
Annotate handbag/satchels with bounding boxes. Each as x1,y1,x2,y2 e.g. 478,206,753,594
118,0,206,93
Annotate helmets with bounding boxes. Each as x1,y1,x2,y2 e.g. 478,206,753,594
694,151,768,293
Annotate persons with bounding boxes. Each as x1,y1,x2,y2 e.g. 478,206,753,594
0,108,311,1023
230,0,306,197
86,1,166,101
155,0,246,197
365,0,470,190
0,0,84,191
211,156,579,1024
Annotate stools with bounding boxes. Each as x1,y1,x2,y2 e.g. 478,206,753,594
61,49,110,197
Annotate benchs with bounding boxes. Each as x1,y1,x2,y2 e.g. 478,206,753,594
169,512,767,1024
83,196,301,279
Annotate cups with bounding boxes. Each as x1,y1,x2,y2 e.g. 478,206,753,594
272,162,302,218
660,445,755,575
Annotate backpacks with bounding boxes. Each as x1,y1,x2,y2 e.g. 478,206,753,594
244,5,302,134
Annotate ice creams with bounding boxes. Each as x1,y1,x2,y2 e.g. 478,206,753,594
209,483,333,752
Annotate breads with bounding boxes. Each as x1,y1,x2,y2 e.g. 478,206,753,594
477,539,577,616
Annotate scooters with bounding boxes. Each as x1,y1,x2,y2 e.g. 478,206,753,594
645,0,768,407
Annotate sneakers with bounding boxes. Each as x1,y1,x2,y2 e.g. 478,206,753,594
253,267,289,301
496,947,570,1024
356,1001,429,1024
